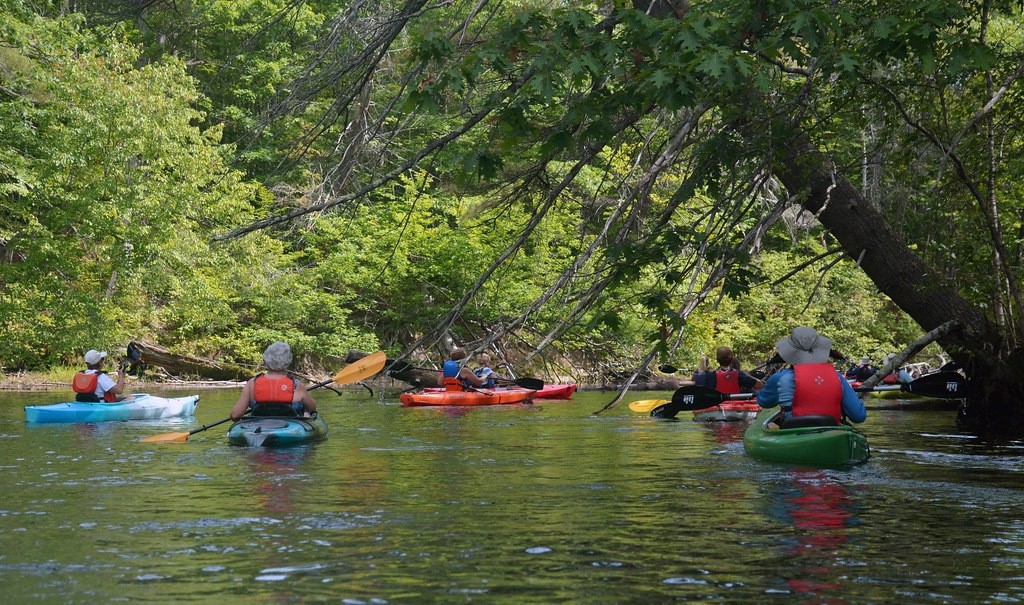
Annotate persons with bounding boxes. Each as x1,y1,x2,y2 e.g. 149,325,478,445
472,353,498,388
691,347,764,394
883,353,919,385
230,341,316,419
73,349,126,402
846,356,877,382
756,326,867,424
438,349,495,391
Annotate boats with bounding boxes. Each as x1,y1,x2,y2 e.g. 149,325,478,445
22,392,201,428
225,406,330,450
398,375,538,409
742,404,870,471
498,377,577,399
692,397,763,424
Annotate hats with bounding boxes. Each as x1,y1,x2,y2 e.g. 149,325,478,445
450,349,467,361
859,356,873,364
477,353,491,365
882,353,897,365
263,341,294,371
775,327,832,365
84,349,108,366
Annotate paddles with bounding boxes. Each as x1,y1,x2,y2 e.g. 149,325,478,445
658,364,766,380
116,341,140,372
650,402,680,418
143,338,418,443
830,349,859,367
384,360,545,390
490,341,517,381
671,370,969,411
628,399,671,413
